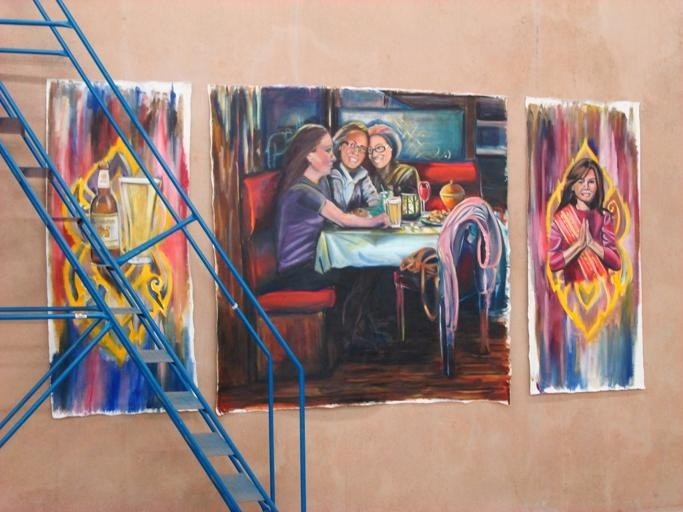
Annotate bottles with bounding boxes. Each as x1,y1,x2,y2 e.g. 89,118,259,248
90,163,121,265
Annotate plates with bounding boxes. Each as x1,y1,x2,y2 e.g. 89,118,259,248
420,212,447,226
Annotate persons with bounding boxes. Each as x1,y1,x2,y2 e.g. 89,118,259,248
364,118,423,197
549,157,622,390
270,123,393,355
326,122,378,211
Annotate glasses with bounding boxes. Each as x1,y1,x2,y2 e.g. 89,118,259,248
342,140,366,153
366,144,390,153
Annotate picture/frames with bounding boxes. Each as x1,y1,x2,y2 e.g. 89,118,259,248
475,118,508,158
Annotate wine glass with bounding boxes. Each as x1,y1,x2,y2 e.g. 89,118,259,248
417,181,432,218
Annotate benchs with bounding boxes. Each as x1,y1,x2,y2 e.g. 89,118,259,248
240,160,478,386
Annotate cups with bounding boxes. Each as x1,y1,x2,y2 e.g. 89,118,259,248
118,176,162,264
402,192,420,220
439,180,465,212
385,197,402,228
